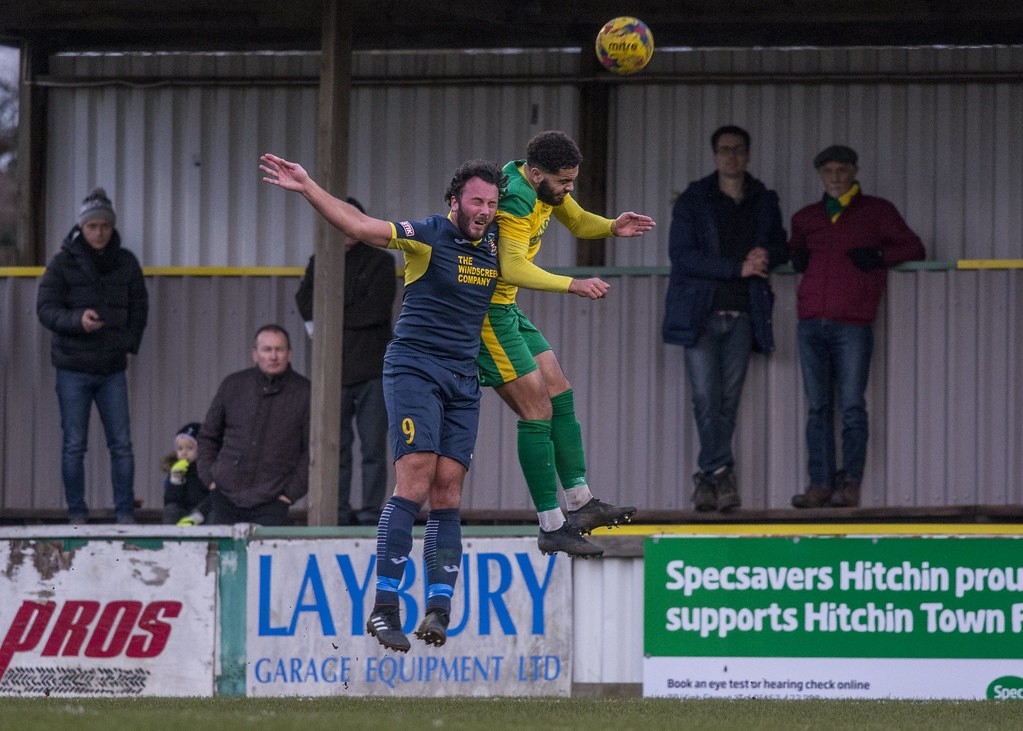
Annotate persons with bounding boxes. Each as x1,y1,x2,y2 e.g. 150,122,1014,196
258,153,501,652
475,131,656,560
36,187,148,524
661,125,788,512
160,422,202,524
197,326,310,525
786,148,926,507
297,198,394,523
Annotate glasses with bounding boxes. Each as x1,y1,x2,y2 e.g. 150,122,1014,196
714,144,750,158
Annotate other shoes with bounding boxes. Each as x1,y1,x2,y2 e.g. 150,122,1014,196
358,509,382,528
338,511,356,527
69,508,88,525
119,510,139,526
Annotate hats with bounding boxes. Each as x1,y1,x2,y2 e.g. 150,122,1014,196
176,423,201,441
813,145,857,169
76,187,116,228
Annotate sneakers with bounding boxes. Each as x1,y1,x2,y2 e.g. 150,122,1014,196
691,471,717,509
566,497,636,535
828,481,859,507
366,610,411,653
713,468,741,511
792,486,834,508
538,522,604,559
414,610,446,647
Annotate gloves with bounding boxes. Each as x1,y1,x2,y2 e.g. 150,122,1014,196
170,460,190,485
845,247,883,273
179,513,204,526
792,249,810,272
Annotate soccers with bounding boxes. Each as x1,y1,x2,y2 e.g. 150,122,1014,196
595,16,654,76
